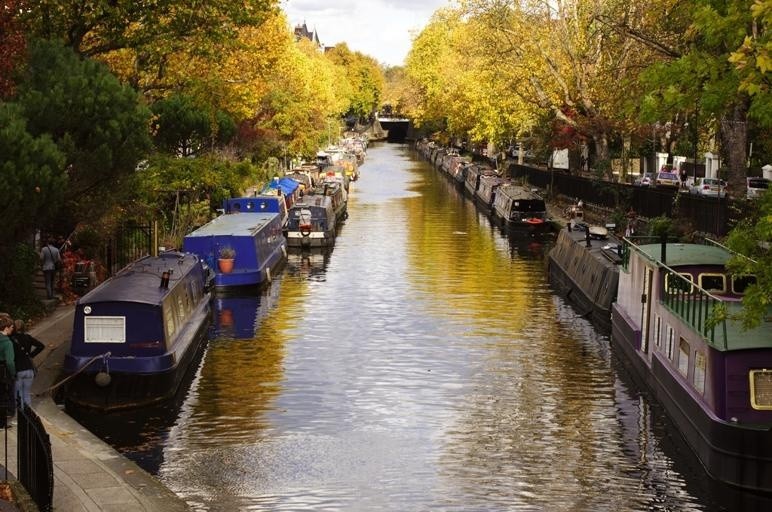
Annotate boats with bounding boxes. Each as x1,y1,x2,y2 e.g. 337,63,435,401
611,337,772,510
182,195,288,290
542,214,665,333
208,272,280,340
60,244,217,428
549,285,614,375
59,338,211,477
415,136,555,241
257,134,368,247
608,229,771,494
286,247,329,272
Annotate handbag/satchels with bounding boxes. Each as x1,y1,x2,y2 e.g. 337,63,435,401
32,366,38,377
55,263,63,272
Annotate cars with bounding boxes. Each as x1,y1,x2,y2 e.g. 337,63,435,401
747,176,771,201
608,169,727,202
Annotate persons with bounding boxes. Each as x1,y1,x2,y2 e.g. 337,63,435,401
10,319,48,428
679,168,689,189
37,236,63,300
0,314,18,431
